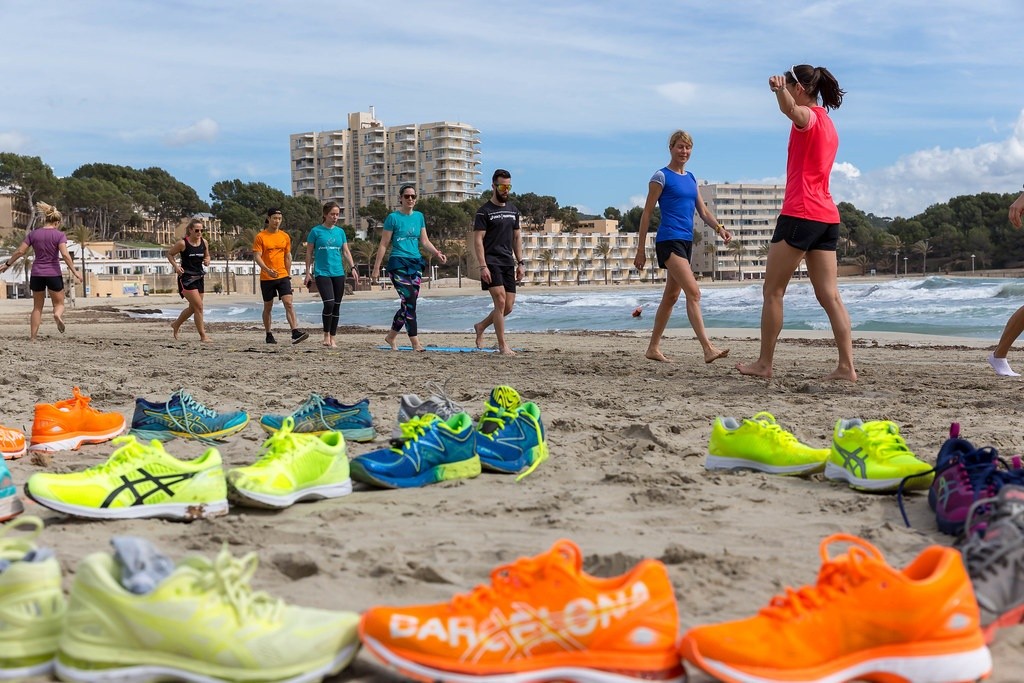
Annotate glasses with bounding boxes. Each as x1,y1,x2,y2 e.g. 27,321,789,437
493,183,512,191
195,229,204,233
403,194,417,199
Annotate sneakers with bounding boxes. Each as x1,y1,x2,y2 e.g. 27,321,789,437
226,415,352,510
127,388,251,446
259,392,378,443
703,411,1024,624
265,334,277,345
360,538,683,683
349,411,481,490
29,387,127,453
392,381,479,442
0,425,27,461
0,513,67,681
681,533,993,683
475,385,549,481
0,453,26,522
54,536,362,683
24,435,231,522
291,332,310,345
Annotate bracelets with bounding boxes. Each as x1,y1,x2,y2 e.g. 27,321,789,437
436,250,442,256
351,266,357,271
715,224,725,233
5,261,11,267
480,264,487,271
518,260,524,265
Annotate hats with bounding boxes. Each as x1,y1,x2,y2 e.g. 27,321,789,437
265,208,283,224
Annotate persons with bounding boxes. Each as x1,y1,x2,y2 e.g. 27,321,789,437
372,184,446,352
734,64,858,382
0,206,83,339
632,308,642,316
988,191,1024,376
634,130,732,363
166,219,214,342
303,201,358,347
474,169,524,355
252,209,309,344
